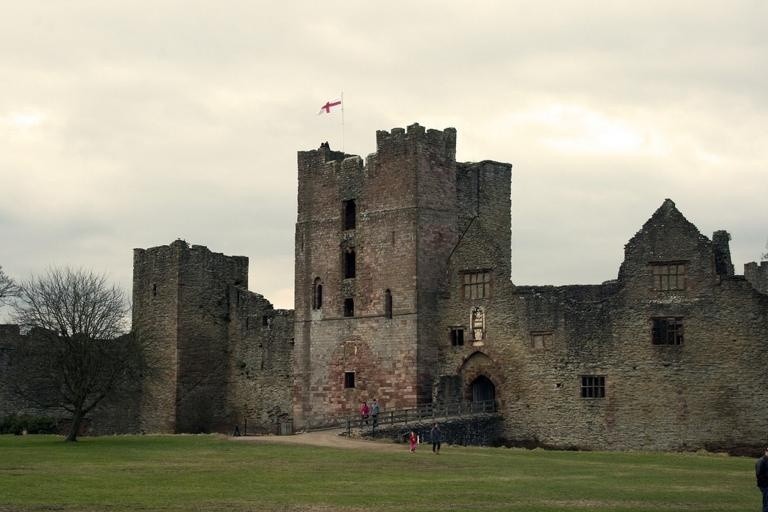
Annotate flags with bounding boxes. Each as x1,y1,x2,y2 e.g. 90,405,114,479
316,91,343,115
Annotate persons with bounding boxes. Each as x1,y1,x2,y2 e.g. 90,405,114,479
431,422,443,456
359,401,370,432
409,431,417,453
755,442,768,512
371,399,379,430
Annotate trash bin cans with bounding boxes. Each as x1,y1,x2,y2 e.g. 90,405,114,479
280,418,293,435
